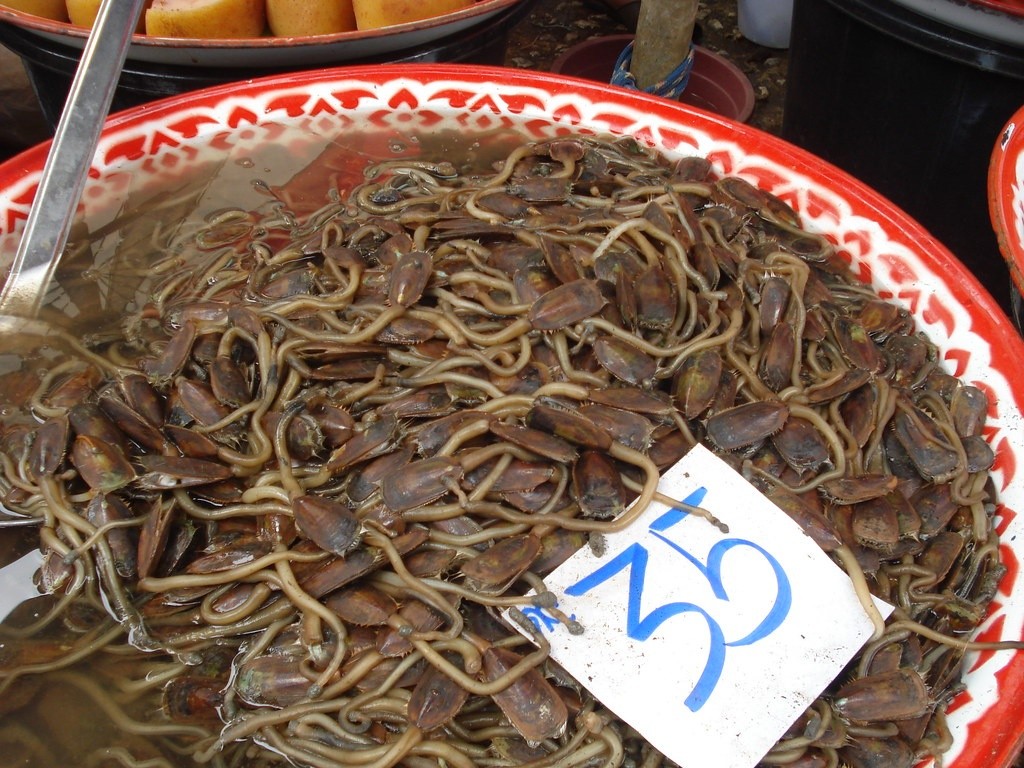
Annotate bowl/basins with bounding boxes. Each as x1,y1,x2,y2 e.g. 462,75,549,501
0,62,1024,768
1,0,514,132
987,106,1024,296
